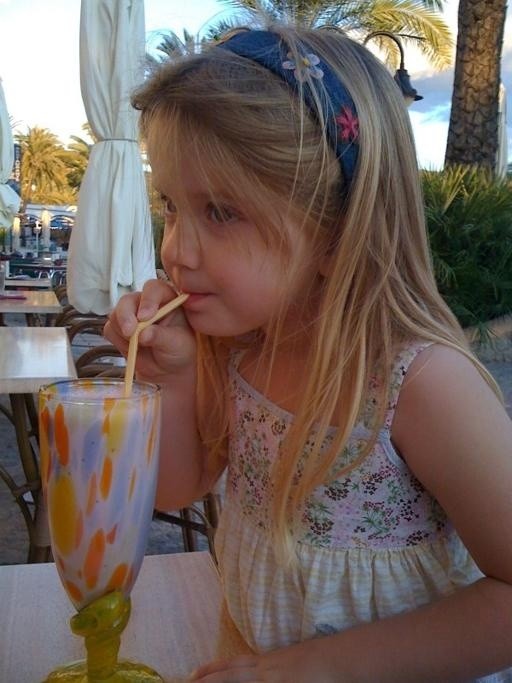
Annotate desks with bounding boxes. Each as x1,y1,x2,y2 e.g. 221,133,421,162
0,277,77,563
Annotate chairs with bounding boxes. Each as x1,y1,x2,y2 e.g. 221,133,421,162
45,279,222,552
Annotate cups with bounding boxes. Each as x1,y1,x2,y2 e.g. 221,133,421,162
52,253,63,266
0,264,6,295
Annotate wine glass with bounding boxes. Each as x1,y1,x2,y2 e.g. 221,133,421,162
39,378,164,683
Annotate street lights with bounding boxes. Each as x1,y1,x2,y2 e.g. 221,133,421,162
362,30,424,114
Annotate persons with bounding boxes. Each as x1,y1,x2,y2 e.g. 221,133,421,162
103,0,512,683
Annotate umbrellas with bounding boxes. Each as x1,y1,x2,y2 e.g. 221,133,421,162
66,0,158,315
0,76,23,230
